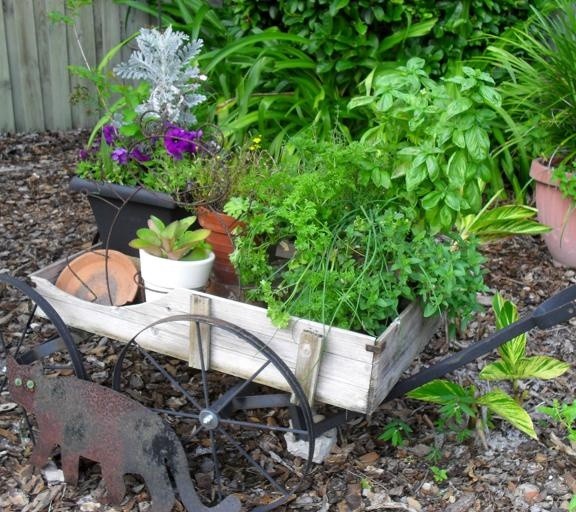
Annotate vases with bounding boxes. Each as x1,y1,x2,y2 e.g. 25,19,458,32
72,179,184,256
198,204,253,286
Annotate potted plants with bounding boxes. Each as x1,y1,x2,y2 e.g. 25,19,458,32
130,215,216,302
456,0,576,269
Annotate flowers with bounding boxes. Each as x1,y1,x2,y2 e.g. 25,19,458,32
50,0,215,194
175,133,275,212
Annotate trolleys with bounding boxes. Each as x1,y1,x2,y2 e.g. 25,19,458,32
0,243,575,507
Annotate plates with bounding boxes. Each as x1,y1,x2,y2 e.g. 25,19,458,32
55,248,138,306
